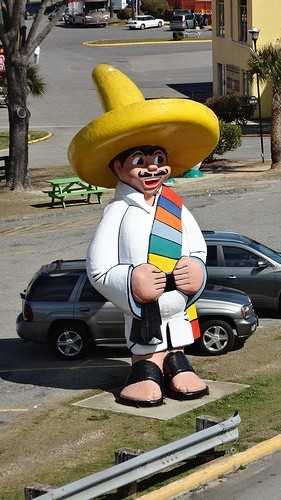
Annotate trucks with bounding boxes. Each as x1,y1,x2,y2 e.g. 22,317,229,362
65,7,111,28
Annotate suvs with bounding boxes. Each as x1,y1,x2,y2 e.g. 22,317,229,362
15,259,259,355
198,230,281,310
170,13,195,31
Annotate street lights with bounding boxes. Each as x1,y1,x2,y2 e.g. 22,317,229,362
248,26,265,164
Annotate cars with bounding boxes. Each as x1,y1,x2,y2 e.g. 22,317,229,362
48,8,65,21
125,15,165,30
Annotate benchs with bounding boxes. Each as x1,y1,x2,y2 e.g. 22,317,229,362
42,187,108,200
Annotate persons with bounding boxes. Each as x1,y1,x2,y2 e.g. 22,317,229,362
33,46,41,63
67,62,220,408
193,13,211,26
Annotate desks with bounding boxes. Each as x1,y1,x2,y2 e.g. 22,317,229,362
44,177,102,209
